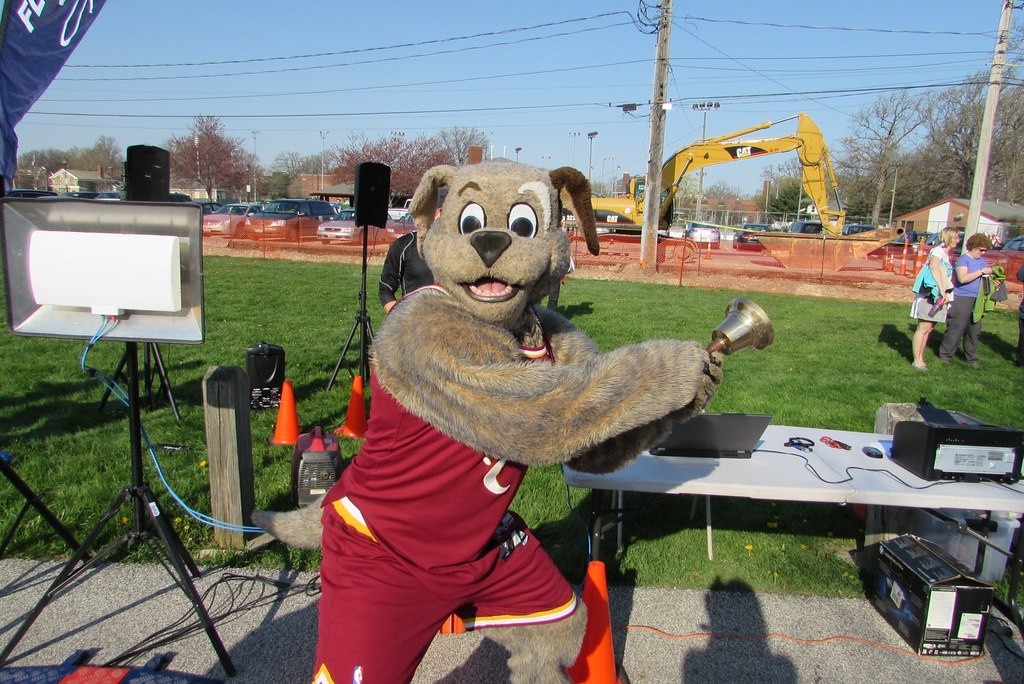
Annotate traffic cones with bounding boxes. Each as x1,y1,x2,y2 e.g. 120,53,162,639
885,253,896,273
562,560,630,684
333,375,367,439
703,243,713,260
270,380,302,445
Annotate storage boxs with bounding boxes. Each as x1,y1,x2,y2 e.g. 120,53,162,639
874,535,994,656
915,507,1020,580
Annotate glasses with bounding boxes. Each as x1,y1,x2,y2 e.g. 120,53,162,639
978,247,988,252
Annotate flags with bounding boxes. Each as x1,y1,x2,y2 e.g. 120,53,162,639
0,0,107,191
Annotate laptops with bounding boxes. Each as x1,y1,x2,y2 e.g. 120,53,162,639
650,413,774,460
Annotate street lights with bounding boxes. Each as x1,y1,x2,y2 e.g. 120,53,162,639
541,156,551,168
252,130,262,202
569,131,598,186
889,162,904,226
320,130,329,190
515,147,521,161
693,101,720,220
601,157,620,197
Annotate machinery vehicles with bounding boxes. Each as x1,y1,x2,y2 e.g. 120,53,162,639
561,110,905,273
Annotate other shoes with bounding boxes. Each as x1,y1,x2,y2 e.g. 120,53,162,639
912,364,927,371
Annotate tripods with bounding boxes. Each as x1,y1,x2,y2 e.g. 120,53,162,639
325,226,375,393
98,341,182,423
0,340,238,678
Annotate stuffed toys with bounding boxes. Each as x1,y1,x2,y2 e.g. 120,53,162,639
251,157,724,684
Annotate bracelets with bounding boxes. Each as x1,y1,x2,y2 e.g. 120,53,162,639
980,268,984,275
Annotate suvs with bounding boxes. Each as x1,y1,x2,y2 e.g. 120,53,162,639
914,231,1008,275
782,220,823,233
682,220,720,250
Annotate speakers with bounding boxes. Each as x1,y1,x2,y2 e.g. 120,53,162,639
125,144,170,203
354,161,391,229
246,344,286,409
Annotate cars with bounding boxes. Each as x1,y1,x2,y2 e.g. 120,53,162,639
732,224,771,251
388,213,417,239
7,190,222,212
999,235,1024,277
843,225,912,259
896,230,935,257
202,203,268,238
658,228,669,238
316,209,393,245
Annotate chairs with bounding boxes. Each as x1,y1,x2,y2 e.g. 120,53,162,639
611,409,713,560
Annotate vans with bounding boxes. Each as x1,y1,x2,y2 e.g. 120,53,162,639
242,200,336,243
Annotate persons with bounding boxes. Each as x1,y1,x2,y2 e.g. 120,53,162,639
939,233,1001,363
378,205,442,313
334,199,341,214
538,258,575,308
910,227,960,368
1016,264,1024,367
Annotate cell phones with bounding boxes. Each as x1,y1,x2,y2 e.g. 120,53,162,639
862,447,883,459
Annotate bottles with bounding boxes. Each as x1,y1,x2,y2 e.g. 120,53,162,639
928,298,945,318
982,275,990,295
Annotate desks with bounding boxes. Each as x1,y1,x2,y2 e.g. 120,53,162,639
563,424,1024,643
